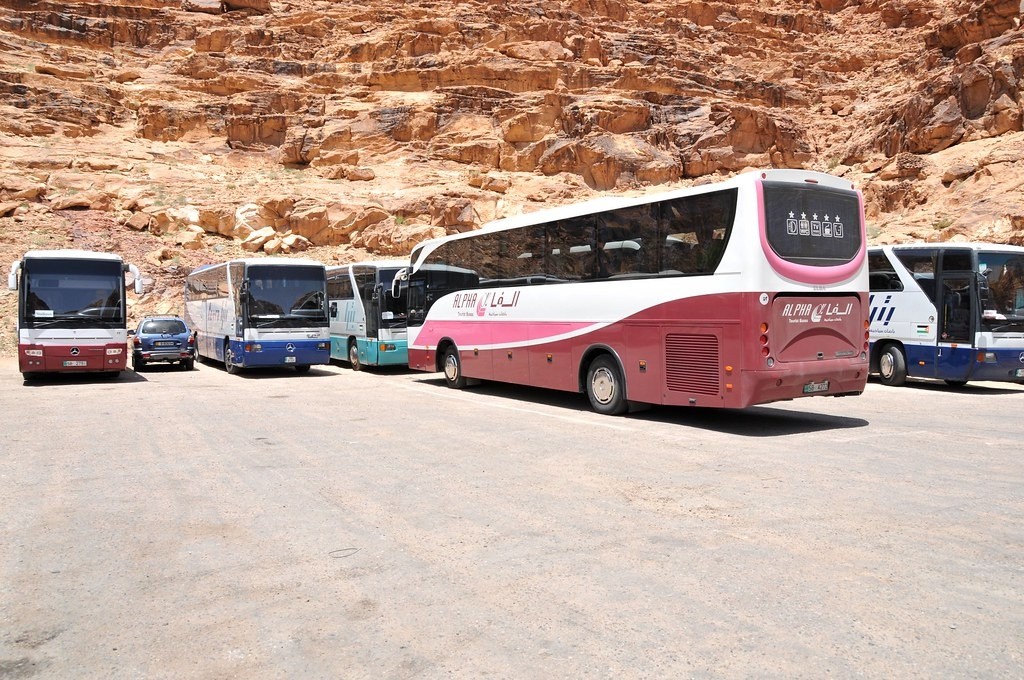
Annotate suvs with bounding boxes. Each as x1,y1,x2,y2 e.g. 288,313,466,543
127,317,197,371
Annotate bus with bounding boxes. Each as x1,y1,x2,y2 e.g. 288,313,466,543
8,249,143,382
323,259,408,371
516,233,691,282
414,263,478,310
481,273,564,290
183,257,338,377
867,240,1024,391
389,169,872,417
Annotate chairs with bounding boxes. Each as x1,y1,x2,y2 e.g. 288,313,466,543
915,272,935,302
696,239,723,272
664,242,691,272
